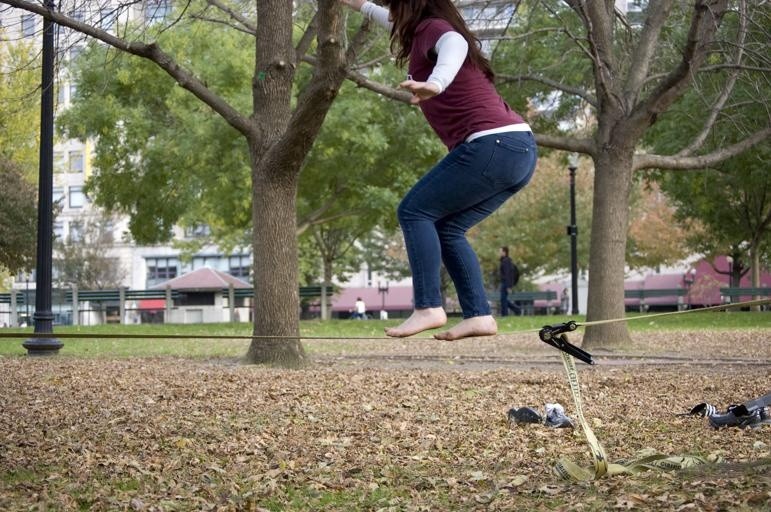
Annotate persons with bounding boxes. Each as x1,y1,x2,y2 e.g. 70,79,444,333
560,286,571,314
355,296,368,320
498,244,525,316
342,1,540,342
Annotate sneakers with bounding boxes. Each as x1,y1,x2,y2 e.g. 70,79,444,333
508,407,543,423
544,403,576,428
709,406,771,430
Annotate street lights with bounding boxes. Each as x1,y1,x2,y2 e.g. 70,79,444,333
565,153,578,316
727,255,732,303
